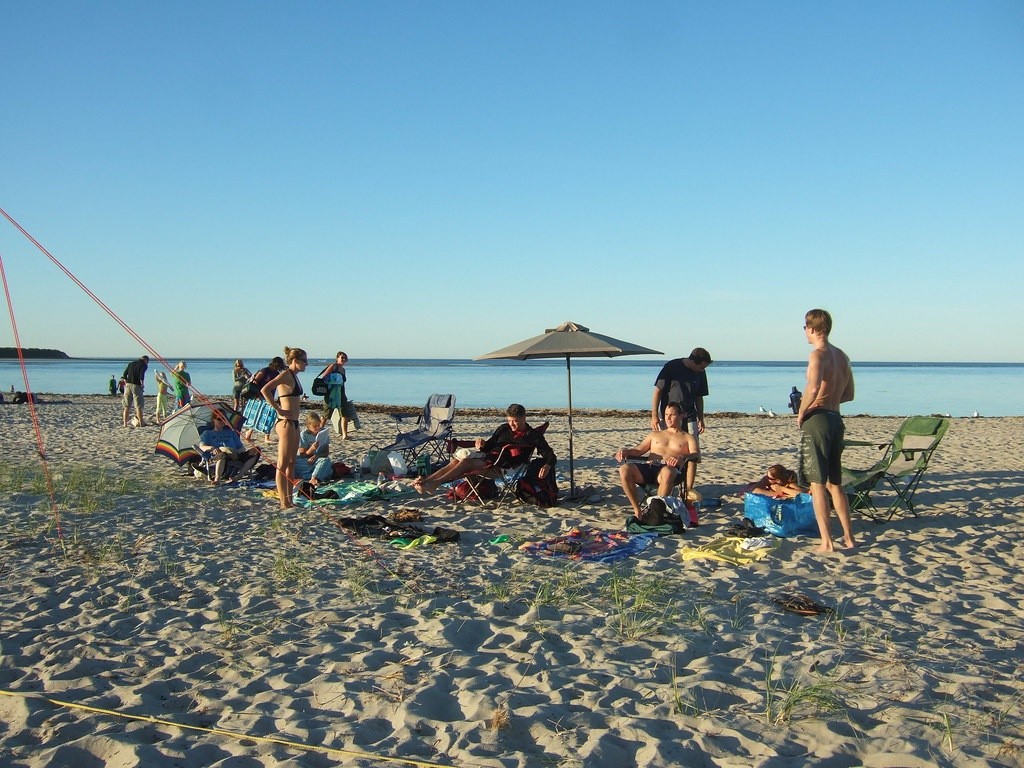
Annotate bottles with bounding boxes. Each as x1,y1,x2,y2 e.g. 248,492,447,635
790,387,802,414
491,535,507,545
506,474,510,481
362,455,368,473
685,499,698,527
693,498,721,506
354,460,360,482
378,472,385,487
559,474,564,482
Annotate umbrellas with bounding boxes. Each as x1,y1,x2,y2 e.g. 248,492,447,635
154,399,247,470
471,321,665,495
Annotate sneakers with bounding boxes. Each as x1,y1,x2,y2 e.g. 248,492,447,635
389,507,424,522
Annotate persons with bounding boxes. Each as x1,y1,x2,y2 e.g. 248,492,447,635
651,348,713,443
410,403,558,496
170,361,191,414
752,464,811,499
10,384,14,393
260,346,309,511
615,402,702,522
314,351,348,440
231,356,287,444
123,355,150,428
109,374,126,397
153,369,174,424
294,410,334,486
796,309,856,552
199,408,261,486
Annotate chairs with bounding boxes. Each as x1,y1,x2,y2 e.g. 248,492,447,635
794,443,894,521
627,454,702,510
198,421,252,482
845,415,949,523
377,393,550,508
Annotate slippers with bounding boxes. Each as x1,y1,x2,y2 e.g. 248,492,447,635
777,593,836,617
426,526,459,545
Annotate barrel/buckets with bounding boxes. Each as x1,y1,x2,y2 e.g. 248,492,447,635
330,400,360,434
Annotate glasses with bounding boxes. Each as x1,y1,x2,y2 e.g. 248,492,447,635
803,326,807,330
297,358,307,363
215,417,228,421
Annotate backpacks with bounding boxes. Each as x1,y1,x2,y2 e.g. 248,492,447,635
517,457,559,507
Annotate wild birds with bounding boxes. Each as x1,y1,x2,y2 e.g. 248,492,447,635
974,410,979,418
945,411,951,416
769,409,778,418
759,405,767,413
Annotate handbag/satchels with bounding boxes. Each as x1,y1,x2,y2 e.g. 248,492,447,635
241,381,258,399
445,476,499,500
312,378,327,396
743,491,820,537
363,445,408,477
241,396,281,433
